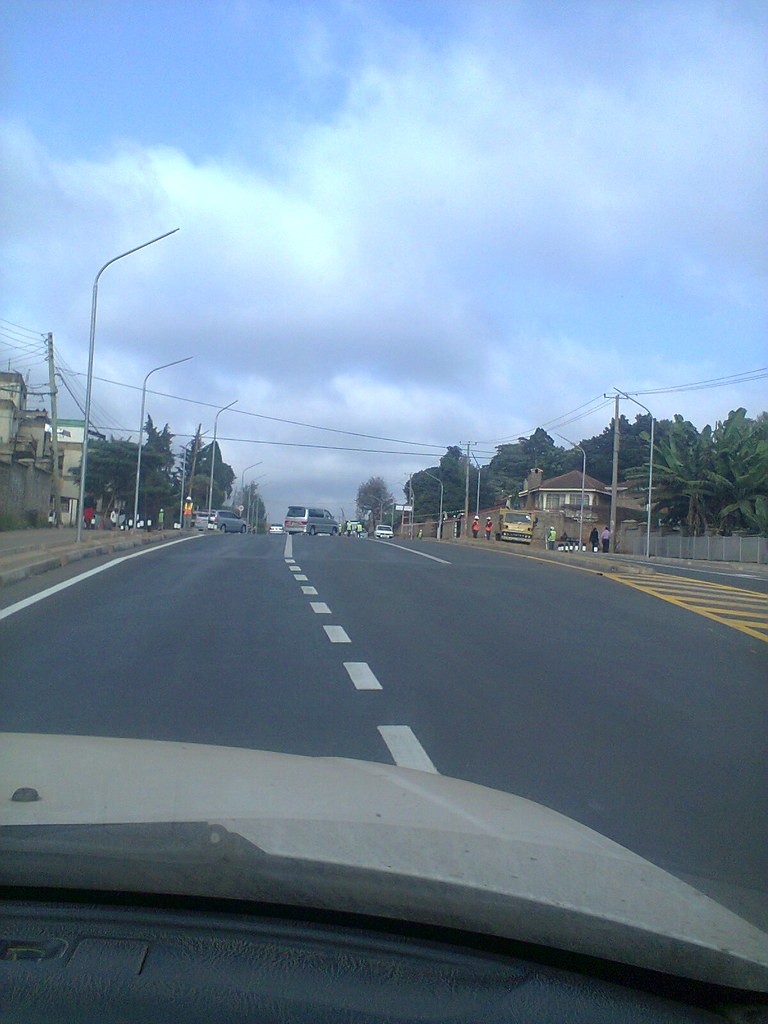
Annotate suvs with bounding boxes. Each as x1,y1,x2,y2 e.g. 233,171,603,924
194,509,247,535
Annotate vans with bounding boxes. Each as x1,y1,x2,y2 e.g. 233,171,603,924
284,505,339,536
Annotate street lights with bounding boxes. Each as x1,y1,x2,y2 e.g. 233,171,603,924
179,428,209,531
77,227,181,538
370,494,383,525
230,460,264,518
472,452,481,517
422,470,443,538
554,432,586,551
613,386,654,557
132,357,194,532
208,399,239,520
399,483,414,541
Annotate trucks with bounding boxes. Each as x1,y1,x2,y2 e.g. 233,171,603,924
495,506,538,545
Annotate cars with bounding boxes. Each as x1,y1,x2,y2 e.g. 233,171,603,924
373,524,394,540
269,522,285,535
348,518,368,540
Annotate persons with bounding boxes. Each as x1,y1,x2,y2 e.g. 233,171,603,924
601,526,611,553
589,528,599,552
183,496,194,529
547,526,556,550
485,516,493,540
346,520,352,536
416,529,423,540
84,502,93,529
472,515,480,538
355,521,362,538
339,522,342,536
560,532,567,541
157,508,164,530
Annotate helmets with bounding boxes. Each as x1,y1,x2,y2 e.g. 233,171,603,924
357,522,360,523
186,497,192,501
339,524,341,526
420,530,422,531
486,517,491,519
474,516,479,519
160,509,164,512
348,520,351,523
550,527,555,530
